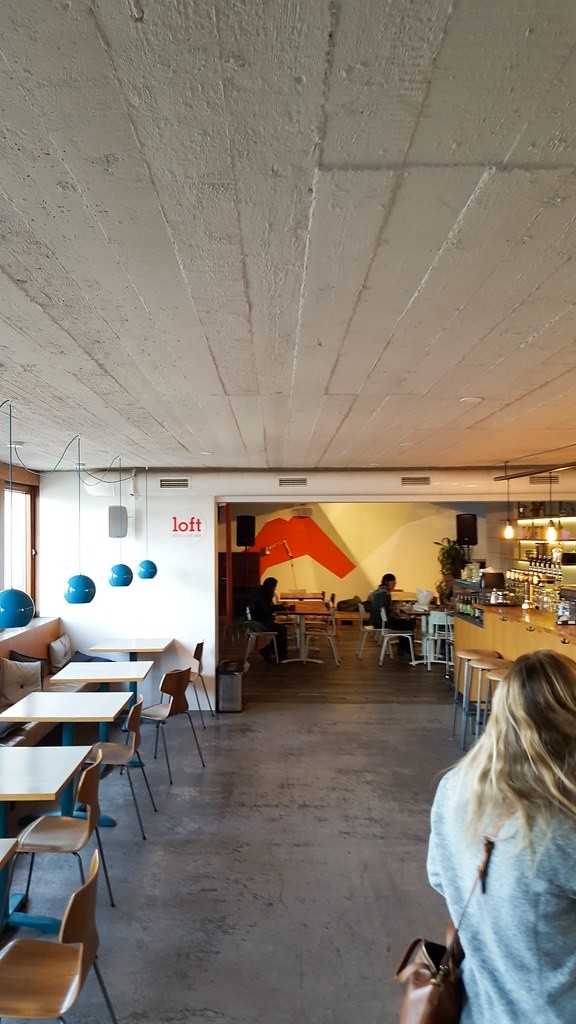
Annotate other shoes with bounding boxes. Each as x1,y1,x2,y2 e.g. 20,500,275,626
259,648,273,663
275,656,291,663
400,651,423,662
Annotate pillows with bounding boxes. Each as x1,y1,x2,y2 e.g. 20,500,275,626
0,633,116,740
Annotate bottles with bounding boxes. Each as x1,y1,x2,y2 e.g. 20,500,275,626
455,556,576,621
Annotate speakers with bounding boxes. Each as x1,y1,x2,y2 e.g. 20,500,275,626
456,513,478,546
237,515,257,549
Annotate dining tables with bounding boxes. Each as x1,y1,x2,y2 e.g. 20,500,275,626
390,606,454,665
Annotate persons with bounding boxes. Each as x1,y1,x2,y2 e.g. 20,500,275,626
250,577,290,662
368,573,421,662
426,650,576,1024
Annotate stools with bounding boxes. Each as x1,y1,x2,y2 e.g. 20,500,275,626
449,649,515,752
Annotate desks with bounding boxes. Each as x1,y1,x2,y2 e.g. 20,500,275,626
280,592,324,639
0,838,61,940
0,691,134,826
276,601,332,664
49,661,154,779
87,638,174,730
0,746,117,850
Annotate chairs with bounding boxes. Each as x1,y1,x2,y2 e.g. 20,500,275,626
243,591,342,666
358,589,454,679
0,642,215,1024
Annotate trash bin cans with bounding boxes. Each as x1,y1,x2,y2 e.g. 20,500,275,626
216,660,244,713
434,624,453,655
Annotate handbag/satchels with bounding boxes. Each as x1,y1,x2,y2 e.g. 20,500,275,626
337,595,361,611
361,601,372,612
393,920,465,1024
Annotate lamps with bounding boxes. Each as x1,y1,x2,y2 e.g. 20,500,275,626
0,398,158,628
